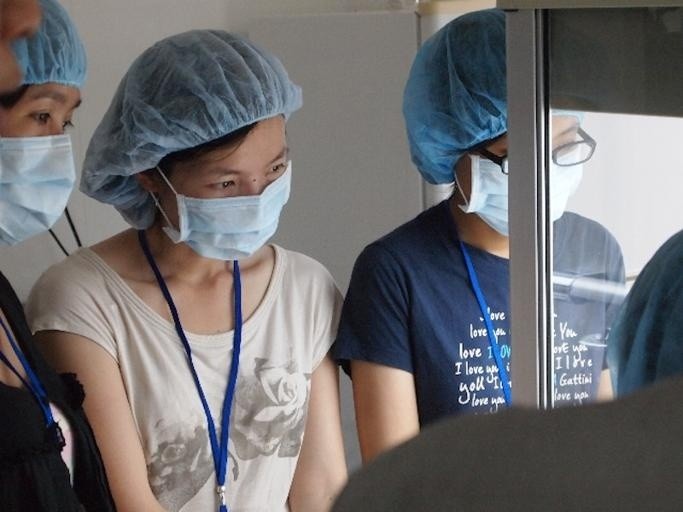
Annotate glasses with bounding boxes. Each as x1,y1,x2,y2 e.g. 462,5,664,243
475,127,596,174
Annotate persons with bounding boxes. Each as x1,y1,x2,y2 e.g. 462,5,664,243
605,227,683,395
329,8,623,468
15,28,352,512
0,0,118,510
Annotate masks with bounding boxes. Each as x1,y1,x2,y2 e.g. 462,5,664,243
150,160,292,261
453,154,582,236
0,135,75,246
